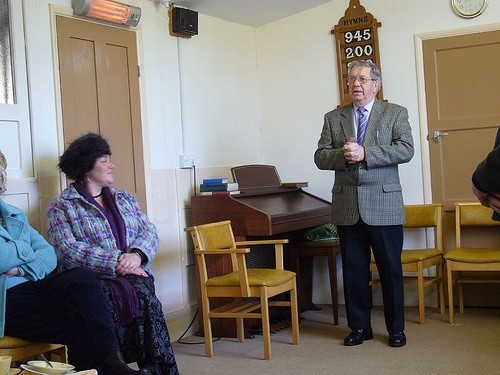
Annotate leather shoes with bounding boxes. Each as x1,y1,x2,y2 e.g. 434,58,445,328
388,331,406,347
344,326,374,346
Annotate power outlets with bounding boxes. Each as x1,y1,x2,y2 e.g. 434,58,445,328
179,154,194,168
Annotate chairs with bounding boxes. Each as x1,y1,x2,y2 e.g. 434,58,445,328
443,202,500,324
370,202,445,324
0,336,69,364
184,220,299,361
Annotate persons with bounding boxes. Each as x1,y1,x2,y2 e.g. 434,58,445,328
0,151,151,375
314,60,414,347
471,125,500,221
45,132,179,375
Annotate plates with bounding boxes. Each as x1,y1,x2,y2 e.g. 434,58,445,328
0,360,74,375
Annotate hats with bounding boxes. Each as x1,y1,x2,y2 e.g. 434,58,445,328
59,133,112,180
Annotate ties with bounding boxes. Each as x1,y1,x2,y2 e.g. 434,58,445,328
357,106,369,145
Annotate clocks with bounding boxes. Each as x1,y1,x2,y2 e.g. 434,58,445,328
452,0,488,19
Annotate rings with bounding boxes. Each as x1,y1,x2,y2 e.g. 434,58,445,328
130,265,134,267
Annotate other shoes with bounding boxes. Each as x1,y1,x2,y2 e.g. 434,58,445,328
97,352,141,375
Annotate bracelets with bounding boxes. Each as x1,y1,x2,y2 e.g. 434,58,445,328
136,251,142,256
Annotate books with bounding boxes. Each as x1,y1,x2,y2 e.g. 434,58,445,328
283,182,309,189
199,178,241,195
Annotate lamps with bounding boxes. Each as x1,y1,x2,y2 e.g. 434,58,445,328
73,0,142,27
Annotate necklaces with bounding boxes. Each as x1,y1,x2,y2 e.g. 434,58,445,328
91,192,102,198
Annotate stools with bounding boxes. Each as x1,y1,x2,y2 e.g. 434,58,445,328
289,240,342,326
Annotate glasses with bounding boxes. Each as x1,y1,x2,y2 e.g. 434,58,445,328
348,76,376,84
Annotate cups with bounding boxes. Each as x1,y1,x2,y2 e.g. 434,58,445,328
0,355,12,375
